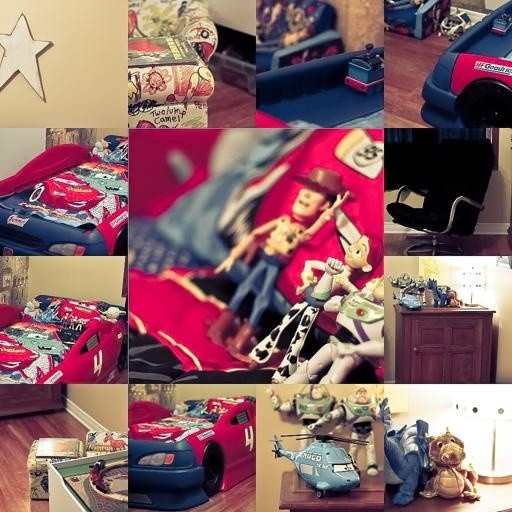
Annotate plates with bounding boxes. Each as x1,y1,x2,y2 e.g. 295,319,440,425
392,303,497,383
44,450,128,512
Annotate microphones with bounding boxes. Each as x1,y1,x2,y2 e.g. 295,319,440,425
86,429,128,459
27,438,83,502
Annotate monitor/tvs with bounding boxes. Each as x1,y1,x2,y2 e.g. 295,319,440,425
36,437,81,459
128,35,201,68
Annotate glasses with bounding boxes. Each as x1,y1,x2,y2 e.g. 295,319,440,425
452,386,511,484
459,265,487,308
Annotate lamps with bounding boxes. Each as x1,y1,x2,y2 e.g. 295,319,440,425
129,0,219,129
385,136,497,255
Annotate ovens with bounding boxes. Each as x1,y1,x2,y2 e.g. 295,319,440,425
0,135,129,256
0,293,128,384
128,394,256,511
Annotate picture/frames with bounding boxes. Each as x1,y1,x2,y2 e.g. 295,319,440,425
386,480,512,512
277,466,385,511
